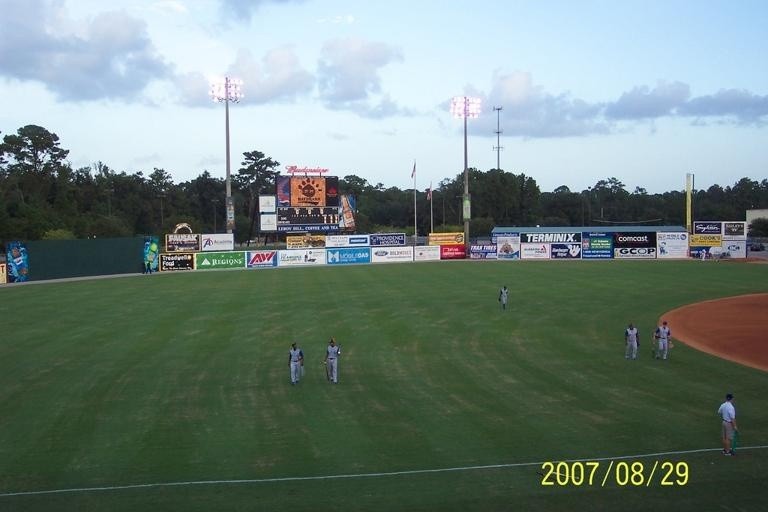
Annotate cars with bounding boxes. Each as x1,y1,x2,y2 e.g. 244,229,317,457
750,243,765,252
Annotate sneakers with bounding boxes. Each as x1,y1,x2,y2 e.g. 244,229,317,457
721,449,737,457
656,353,667,360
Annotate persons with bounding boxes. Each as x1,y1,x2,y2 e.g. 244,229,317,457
702,247,707,261
324,338,340,384
653,321,671,360
718,394,738,456
141,262,146,274
624,324,639,360
288,343,304,385
146,261,151,274
499,286,508,310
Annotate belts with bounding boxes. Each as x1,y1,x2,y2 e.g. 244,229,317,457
722,418,733,423
658,336,668,339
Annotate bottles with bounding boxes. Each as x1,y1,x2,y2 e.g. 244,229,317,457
340,195,354,227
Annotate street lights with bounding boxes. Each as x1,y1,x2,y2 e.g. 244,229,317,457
450,94,481,259
157,194,166,226
209,76,245,236
211,199,219,233
